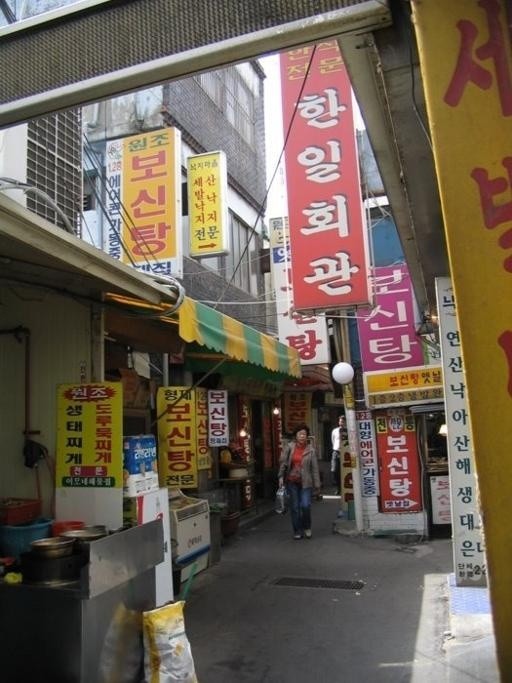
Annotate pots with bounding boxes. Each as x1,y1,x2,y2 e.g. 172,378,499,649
28,527,108,560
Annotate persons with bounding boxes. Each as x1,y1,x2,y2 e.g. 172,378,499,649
278,424,320,539
330,414,346,495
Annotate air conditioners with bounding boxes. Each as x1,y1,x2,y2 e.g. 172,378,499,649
0,106,87,237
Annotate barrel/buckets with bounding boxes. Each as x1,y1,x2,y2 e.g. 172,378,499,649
51,521,84,537
1,517,51,558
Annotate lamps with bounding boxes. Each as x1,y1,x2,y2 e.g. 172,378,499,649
437,413,448,437
332,362,365,404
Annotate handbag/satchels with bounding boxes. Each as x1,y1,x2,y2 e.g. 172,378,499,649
276,485,288,517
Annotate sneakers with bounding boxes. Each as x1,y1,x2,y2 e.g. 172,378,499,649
304,529,312,539
294,531,303,539
